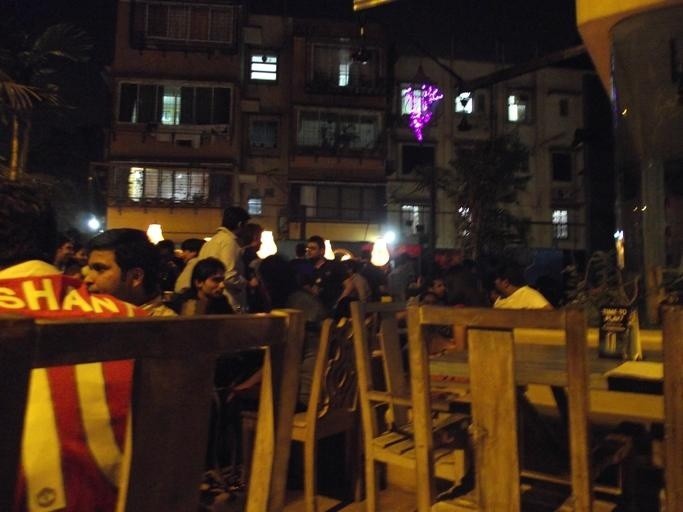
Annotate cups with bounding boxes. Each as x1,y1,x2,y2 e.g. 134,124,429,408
164,291,174,301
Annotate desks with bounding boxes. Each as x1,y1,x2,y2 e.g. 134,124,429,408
428,328,666,466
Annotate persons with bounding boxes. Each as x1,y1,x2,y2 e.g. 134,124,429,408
59,207,573,511
1,179,153,509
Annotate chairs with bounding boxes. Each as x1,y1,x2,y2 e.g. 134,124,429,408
406,305,622,512
0,309,307,512
239,318,370,511
351,300,463,512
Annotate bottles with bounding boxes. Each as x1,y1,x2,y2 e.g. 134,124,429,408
621,308,642,363
